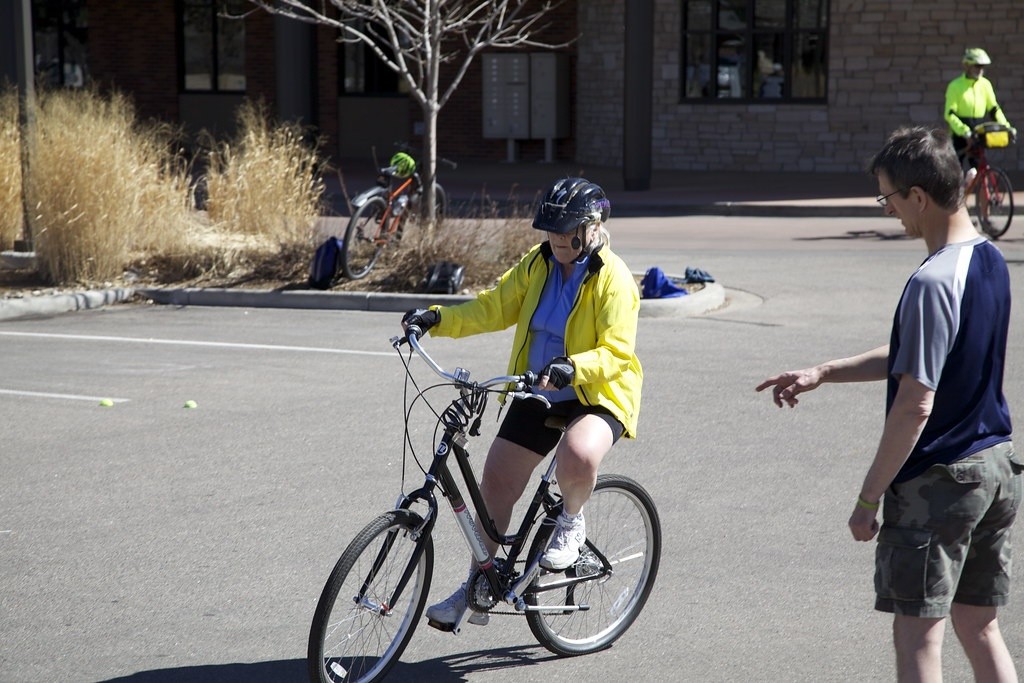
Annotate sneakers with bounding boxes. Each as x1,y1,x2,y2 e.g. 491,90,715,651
426,581,471,624
539,509,586,569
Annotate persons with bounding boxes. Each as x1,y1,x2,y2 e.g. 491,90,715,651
944,48,1015,235
401,178,643,625
756,128,1024,683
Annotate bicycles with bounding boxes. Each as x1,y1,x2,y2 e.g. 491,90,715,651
340,141,460,281
308,308,664,683
946,121,1019,236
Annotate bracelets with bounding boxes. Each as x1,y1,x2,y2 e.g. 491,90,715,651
858,494,879,510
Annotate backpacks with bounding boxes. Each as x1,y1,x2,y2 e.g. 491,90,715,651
640,268,686,298
684,268,713,284
415,261,466,294
308,236,341,290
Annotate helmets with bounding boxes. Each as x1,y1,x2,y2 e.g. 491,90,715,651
533,177,610,232
961,47,992,66
390,152,416,177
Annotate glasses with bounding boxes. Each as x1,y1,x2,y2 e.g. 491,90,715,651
877,185,913,206
